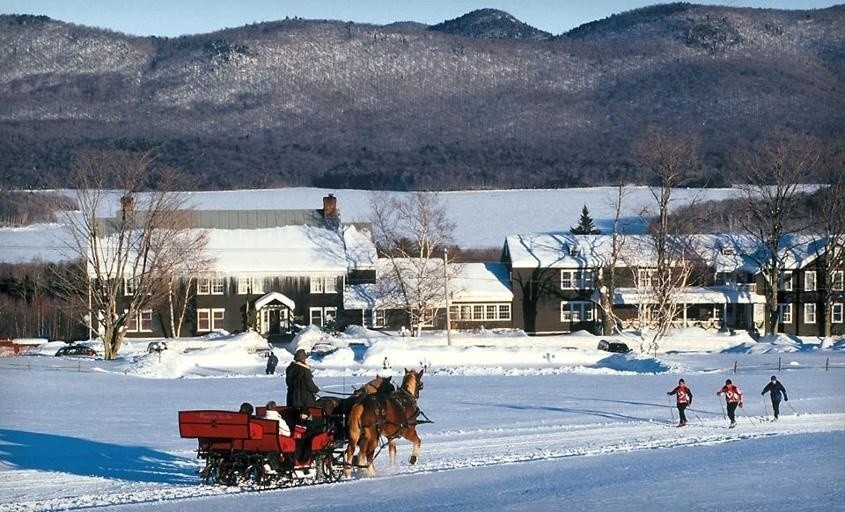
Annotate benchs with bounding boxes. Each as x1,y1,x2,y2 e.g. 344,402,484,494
201,406,322,453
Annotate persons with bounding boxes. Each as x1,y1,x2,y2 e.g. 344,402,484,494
236,400,253,418
264,351,278,374
717,378,745,428
289,408,321,461
283,347,323,408
382,356,392,369
666,378,693,426
261,400,289,434
760,375,790,422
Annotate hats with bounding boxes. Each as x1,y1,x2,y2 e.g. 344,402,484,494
294,349,307,360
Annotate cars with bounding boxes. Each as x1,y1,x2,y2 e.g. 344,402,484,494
54,345,98,357
147,342,167,354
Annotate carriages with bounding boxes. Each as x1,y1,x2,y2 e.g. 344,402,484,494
176,367,434,491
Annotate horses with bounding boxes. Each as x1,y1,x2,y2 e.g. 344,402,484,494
317,374,396,470
344,367,425,479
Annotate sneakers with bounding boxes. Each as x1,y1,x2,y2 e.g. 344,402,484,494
676,421,688,427
728,421,737,428
771,416,778,422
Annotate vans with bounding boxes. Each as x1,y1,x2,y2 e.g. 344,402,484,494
598,339,630,354
